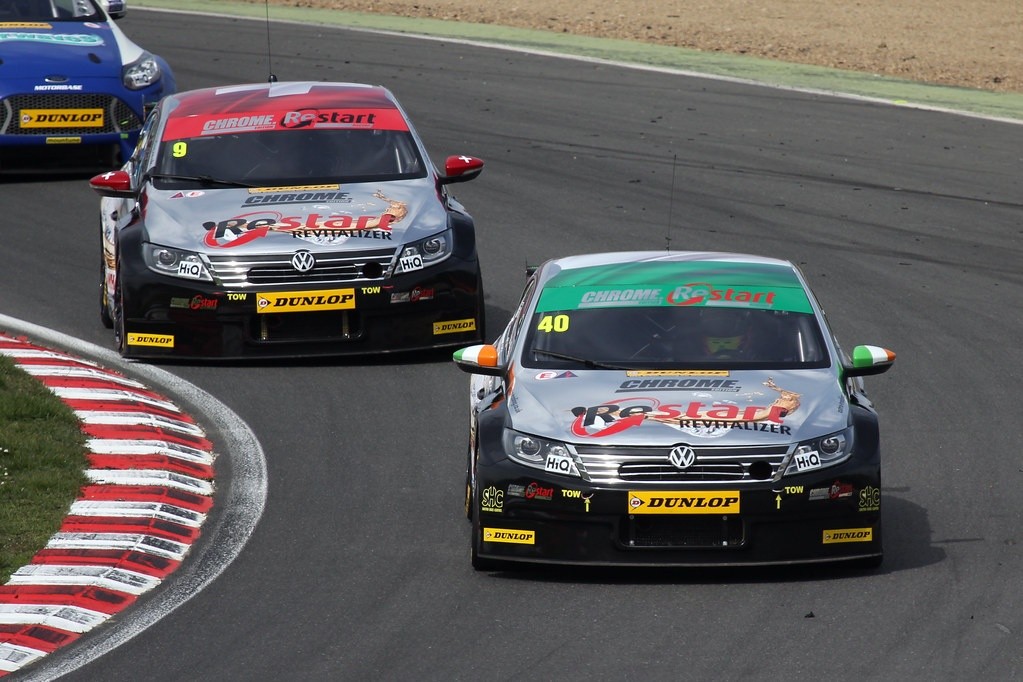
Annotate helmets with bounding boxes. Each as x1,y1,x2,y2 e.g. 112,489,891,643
697,306,749,359
347,126,391,161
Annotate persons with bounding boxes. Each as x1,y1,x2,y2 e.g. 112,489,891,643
704,319,767,361
326,130,395,176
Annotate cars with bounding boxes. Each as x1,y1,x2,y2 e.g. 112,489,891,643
0,0,173,150
452,253,897,572
86,81,486,362
